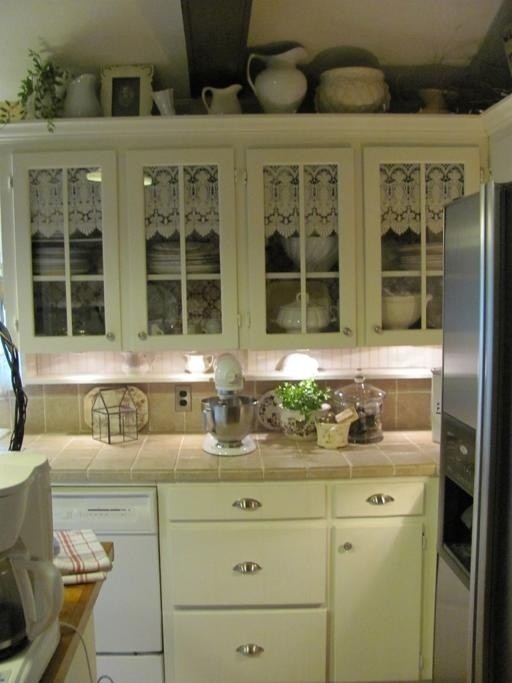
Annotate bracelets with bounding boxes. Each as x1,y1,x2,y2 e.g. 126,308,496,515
383,292,433,328
281,235,339,271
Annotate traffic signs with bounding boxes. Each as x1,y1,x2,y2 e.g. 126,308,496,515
175,386,190,412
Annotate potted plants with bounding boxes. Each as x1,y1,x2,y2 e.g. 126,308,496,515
100,64,155,115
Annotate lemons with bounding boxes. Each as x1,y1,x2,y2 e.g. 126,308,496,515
267,281,331,332
200,85,244,113
64,74,99,119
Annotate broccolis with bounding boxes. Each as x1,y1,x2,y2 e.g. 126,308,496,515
431,183,511,681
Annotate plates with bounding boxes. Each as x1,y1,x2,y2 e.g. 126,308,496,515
0,133,240,350
239,134,482,347
482,121,512,184
158,476,438,682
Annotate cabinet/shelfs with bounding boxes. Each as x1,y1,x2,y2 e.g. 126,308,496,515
333,375,387,443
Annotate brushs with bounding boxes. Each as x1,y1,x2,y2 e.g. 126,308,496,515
1,450,63,683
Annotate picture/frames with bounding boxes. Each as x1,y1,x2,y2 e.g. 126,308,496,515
39,543,113,683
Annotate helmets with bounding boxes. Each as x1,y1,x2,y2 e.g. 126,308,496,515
39,250,89,274
151,245,220,278
397,243,444,273
147,284,178,334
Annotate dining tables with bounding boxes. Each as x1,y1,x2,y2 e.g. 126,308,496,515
277,380,331,439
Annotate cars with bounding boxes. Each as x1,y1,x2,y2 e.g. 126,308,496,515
316,421,351,450
150,88,175,115
244,46,311,113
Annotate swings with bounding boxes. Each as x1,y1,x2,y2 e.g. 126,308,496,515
199,356,262,456
